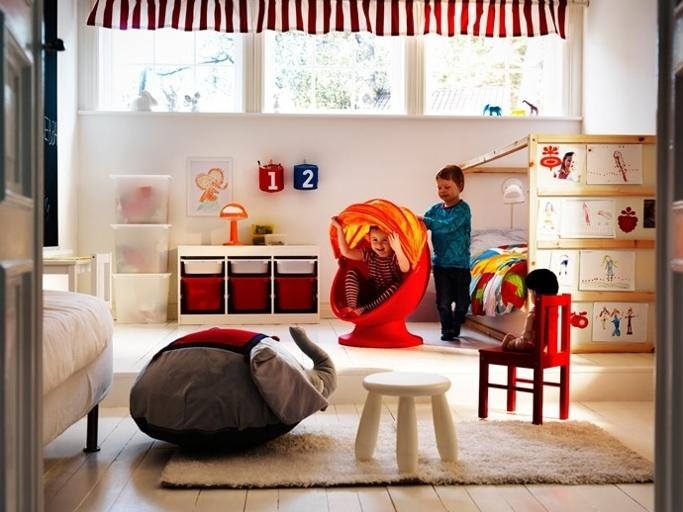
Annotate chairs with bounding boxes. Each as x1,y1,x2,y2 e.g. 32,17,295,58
478,292,572,423
331,241,432,348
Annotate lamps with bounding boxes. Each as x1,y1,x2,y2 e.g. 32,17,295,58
219,201,252,245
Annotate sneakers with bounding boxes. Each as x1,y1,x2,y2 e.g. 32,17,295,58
441,322,461,341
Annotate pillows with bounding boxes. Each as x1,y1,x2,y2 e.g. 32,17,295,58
469,228,518,258
505,225,529,244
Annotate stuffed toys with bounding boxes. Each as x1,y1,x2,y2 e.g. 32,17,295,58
132,327,335,454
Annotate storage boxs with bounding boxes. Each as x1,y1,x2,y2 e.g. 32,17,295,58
228,258,271,275
181,275,224,311
112,173,174,224
275,258,318,275
181,257,223,274
110,225,172,274
274,278,316,312
230,277,270,311
110,274,171,326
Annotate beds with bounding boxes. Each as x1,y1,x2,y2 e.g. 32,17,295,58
42,289,115,464
455,134,659,355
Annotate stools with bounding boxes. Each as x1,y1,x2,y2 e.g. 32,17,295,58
355,370,458,473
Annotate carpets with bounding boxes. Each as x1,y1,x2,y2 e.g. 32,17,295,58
158,417,656,489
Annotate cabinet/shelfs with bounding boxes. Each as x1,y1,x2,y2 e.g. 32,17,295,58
177,245,321,325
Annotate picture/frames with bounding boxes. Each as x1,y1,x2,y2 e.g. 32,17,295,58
186,155,234,220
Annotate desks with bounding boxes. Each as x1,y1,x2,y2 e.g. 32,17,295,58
43,256,97,295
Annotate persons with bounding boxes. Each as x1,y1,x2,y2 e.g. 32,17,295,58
503,269,558,352
332,216,410,316
558,152,574,179
416,164,471,340
